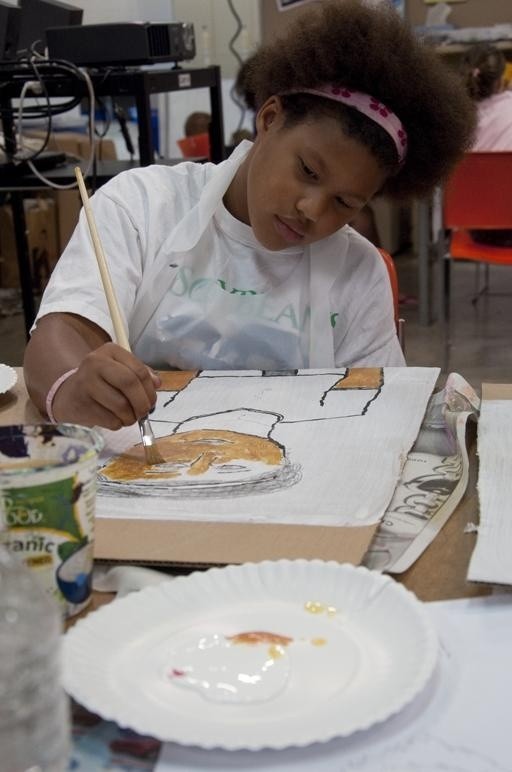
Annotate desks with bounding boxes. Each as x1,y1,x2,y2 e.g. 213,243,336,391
0,366,512,772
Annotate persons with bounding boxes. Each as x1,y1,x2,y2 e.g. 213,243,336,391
23,1,479,437
458,41,512,249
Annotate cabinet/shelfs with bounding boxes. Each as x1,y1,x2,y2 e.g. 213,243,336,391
0,65,224,345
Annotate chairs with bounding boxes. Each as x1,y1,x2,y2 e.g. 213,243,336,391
378,248,406,361
442,152,512,321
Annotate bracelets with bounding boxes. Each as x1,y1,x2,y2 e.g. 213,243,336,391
46,366,80,427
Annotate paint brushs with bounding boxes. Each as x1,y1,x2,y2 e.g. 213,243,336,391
72,166,168,466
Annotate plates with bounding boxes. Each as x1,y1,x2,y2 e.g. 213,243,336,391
0,363,19,396
63,557,438,754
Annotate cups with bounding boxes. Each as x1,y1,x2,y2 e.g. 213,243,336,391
0,423,102,772
